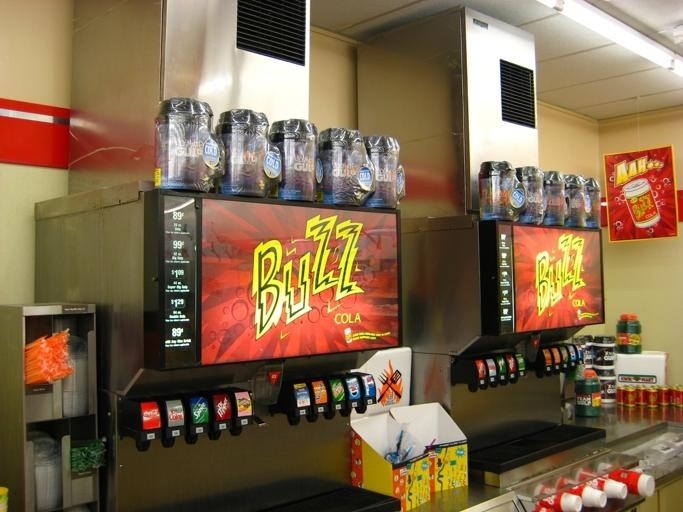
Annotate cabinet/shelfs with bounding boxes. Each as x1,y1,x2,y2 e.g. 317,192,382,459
0,304,104,511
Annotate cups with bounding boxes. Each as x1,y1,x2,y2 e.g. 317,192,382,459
531,462,657,512
152,95,400,211
477,160,602,230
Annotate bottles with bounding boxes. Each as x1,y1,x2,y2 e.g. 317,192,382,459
577,334,617,404
615,313,643,355
576,364,602,418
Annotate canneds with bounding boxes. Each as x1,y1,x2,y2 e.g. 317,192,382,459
616,383,683,409
618,405,683,426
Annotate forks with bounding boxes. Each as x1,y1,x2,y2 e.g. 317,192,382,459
377,373,402,399
